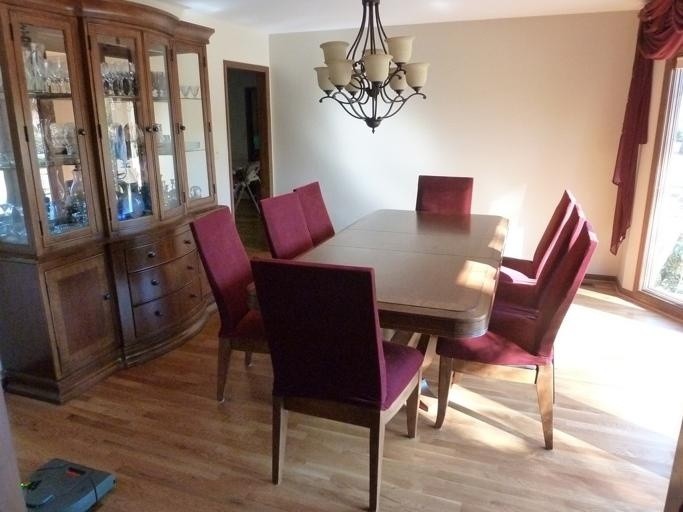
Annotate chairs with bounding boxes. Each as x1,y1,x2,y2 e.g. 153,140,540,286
260,192,313,259
292,181,335,248
250,256,424,510
416,175,474,214
434,222,597,449
232,162,260,216
191,206,268,405
500,190,575,285
492,206,585,383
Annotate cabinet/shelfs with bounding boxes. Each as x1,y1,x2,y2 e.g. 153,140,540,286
0,0,219,405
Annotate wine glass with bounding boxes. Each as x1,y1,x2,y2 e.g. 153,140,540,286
0,41,217,242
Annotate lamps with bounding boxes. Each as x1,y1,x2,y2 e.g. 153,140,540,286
314,0,430,133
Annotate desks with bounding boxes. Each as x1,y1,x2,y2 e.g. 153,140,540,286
290,209,510,412
